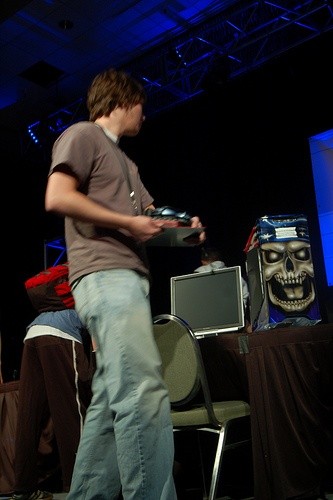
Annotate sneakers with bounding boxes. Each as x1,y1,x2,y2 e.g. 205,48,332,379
13,489,53,500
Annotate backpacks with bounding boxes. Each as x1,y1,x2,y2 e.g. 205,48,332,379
24,261,74,311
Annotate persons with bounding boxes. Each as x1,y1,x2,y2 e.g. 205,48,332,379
194,245,250,310
12,306,93,500
46,68,206,500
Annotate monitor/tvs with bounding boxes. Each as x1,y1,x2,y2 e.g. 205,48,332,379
170,265,245,338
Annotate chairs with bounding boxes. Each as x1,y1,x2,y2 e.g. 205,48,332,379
153,314,251,500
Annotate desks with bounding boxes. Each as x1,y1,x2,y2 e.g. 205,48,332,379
0,324,333,500
